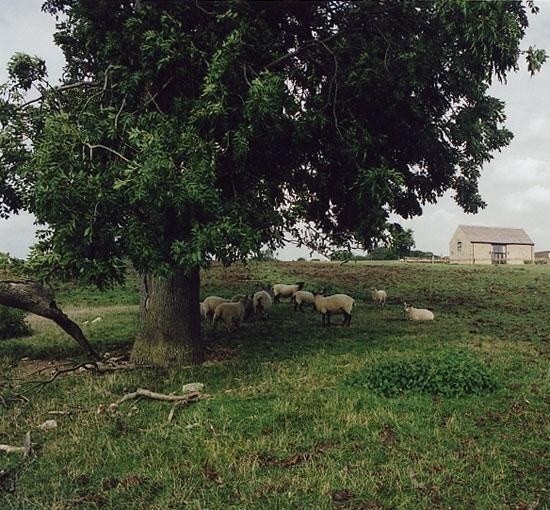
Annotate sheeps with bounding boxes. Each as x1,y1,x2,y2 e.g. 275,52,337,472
289,290,325,314
199,289,272,339
403,301,435,322
310,288,356,327
369,287,387,308
270,281,305,304
